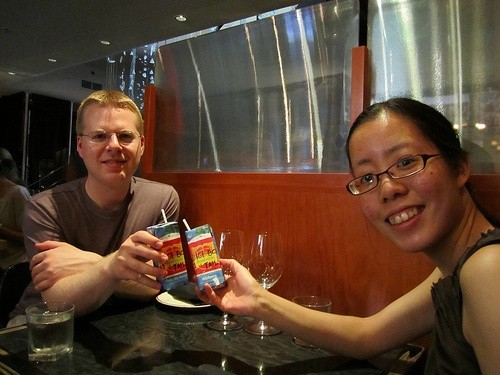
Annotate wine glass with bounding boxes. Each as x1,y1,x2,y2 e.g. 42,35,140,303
242,230,281,336
207,229,243,332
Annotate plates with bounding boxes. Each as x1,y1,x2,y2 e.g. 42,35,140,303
155,292,212,308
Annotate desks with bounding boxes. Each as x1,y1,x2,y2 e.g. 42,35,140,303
0,303,426,375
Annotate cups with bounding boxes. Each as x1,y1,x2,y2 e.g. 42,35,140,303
25,301,75,362
291,296,332,348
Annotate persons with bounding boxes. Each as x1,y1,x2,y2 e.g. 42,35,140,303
0,147,33,329
194,97,500,375
8,89,180,321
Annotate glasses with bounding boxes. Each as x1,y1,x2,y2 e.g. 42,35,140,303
77,132,141,145
347,152,447,195
